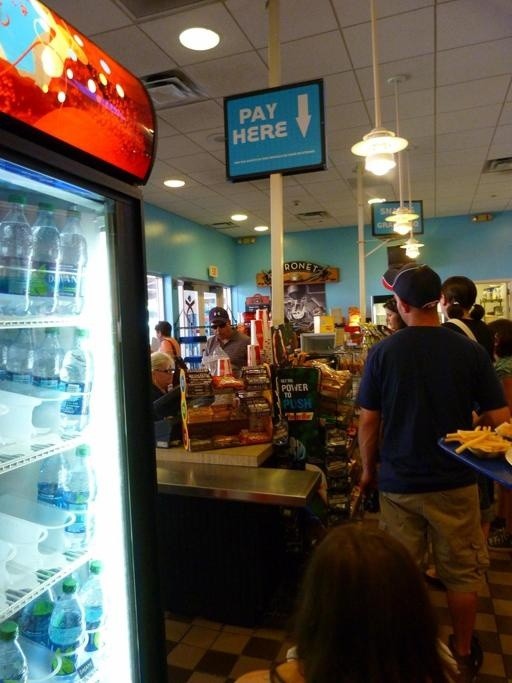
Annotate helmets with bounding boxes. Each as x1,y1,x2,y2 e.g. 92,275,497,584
503,450,512,466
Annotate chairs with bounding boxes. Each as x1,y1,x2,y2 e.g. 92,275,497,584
425,566,441,582
447,632,484,674
486,527,512,551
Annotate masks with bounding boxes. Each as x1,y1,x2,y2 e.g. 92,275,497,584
444,425,508,454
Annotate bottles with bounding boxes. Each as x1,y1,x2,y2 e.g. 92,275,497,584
380,261,442,309
0,327,91,438
0,555,99,682
32,450,96,554
207,307,228,324
1,186,88,318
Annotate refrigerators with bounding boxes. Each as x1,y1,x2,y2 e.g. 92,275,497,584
0,2,171,682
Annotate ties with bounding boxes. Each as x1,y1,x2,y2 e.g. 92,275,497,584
301,334,335,356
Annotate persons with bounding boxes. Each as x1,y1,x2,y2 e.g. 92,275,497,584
355,258,512,681
150,349,174,401
381,302,404,329
203,306,250,366
487,317,512,411
231,519,455,682
441,278,491,544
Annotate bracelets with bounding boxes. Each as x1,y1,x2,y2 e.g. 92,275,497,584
458,435,510,459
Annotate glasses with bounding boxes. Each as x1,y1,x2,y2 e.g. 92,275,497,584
351,0,425,261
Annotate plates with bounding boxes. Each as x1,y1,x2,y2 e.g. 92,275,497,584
503,450,512,466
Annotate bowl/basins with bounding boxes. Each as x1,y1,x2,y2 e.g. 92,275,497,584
458,435,510,459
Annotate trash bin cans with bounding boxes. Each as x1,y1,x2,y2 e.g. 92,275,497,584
155,319,181,358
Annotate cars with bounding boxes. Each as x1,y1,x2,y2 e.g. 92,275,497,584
217,358,233,376
245,308,273,366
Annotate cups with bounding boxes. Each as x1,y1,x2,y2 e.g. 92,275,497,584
153,369,176,375
210,321,227,330
245,308,273,366
217,358,233,376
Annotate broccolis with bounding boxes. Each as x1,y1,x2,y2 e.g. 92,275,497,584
0,2,171,682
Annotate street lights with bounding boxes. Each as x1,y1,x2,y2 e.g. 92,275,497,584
173,355,188,387
